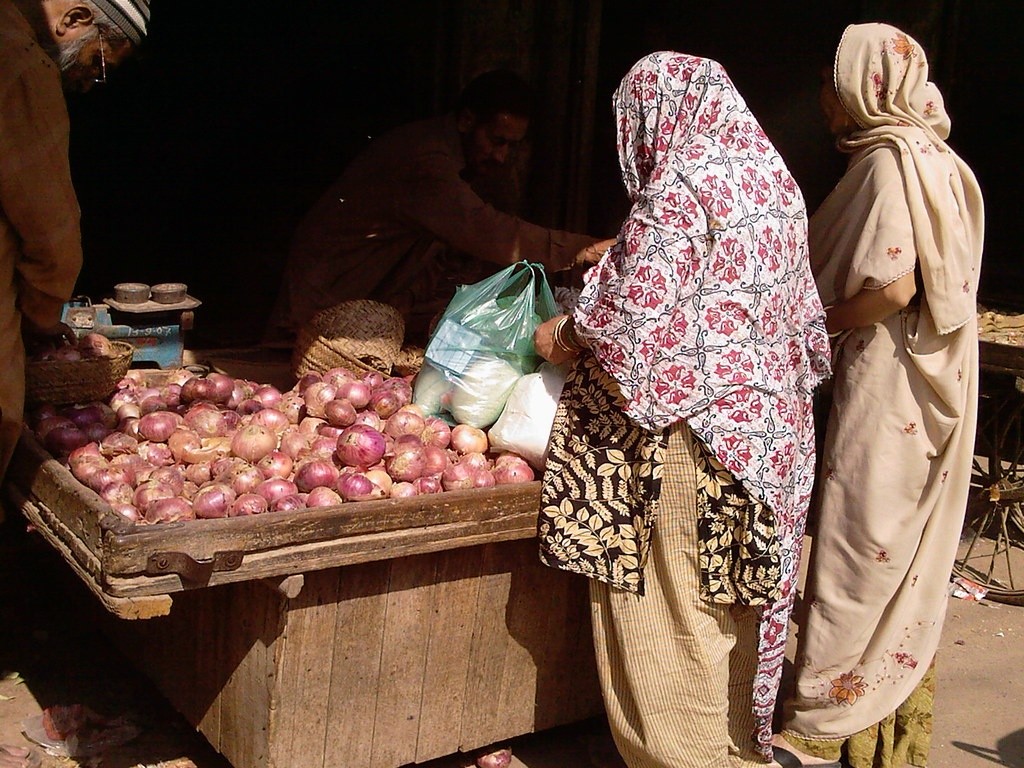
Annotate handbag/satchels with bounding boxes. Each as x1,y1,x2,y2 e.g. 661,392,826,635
413,261,560,428
484,358,573,471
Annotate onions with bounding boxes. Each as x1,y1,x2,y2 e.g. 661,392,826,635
476,747,511,768
23,332,537,524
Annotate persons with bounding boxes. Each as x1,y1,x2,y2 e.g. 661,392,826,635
533,50,834,767
263,69,617,350
0,0,152,527
772,23,987,766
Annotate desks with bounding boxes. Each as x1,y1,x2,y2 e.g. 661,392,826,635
959,364,1024,591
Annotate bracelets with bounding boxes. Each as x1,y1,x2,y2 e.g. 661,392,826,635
554,314,586,352
821,305,843,338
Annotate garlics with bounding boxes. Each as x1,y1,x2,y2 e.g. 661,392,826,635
552,285,582,314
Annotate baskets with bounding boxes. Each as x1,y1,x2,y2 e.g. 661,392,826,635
290,300,426,382
24,341,135,405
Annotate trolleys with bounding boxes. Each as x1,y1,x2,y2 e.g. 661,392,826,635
947,299,1024,608
